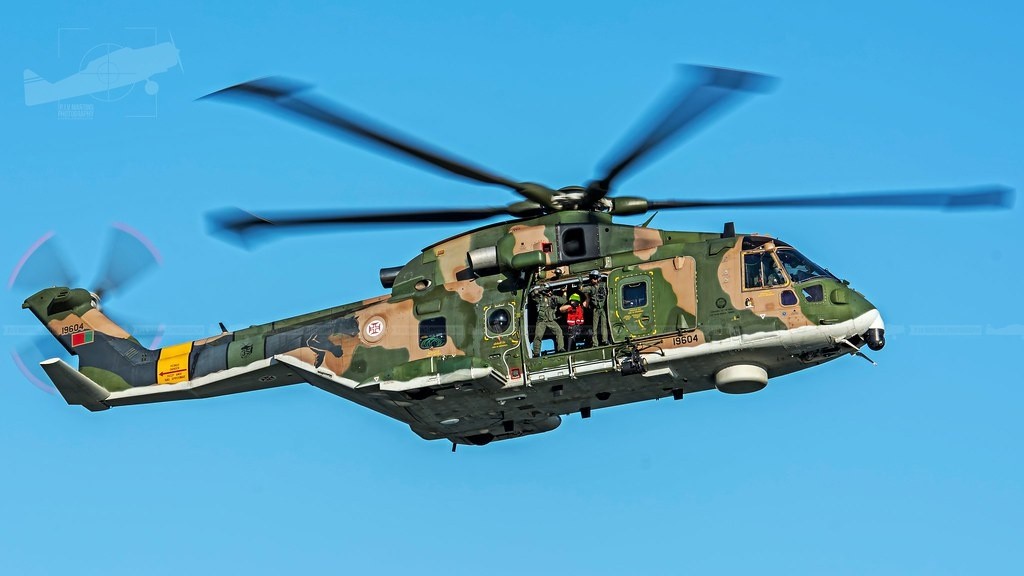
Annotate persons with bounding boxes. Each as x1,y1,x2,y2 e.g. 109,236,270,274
559,292,591,351
767,260,784,285
577,270,609,347
531,282,567,357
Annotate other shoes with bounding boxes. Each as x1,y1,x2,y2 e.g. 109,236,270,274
559,348,566,353
533,354,540,358
603,341,610,346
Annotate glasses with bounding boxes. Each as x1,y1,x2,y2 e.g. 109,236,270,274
589,275,601,280
544,288,553,293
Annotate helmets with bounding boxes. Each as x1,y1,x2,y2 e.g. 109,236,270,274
540,282,553,290
569,293,580,302
589,270,601,281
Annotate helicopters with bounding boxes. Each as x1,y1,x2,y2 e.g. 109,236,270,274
20,60,1019,447
